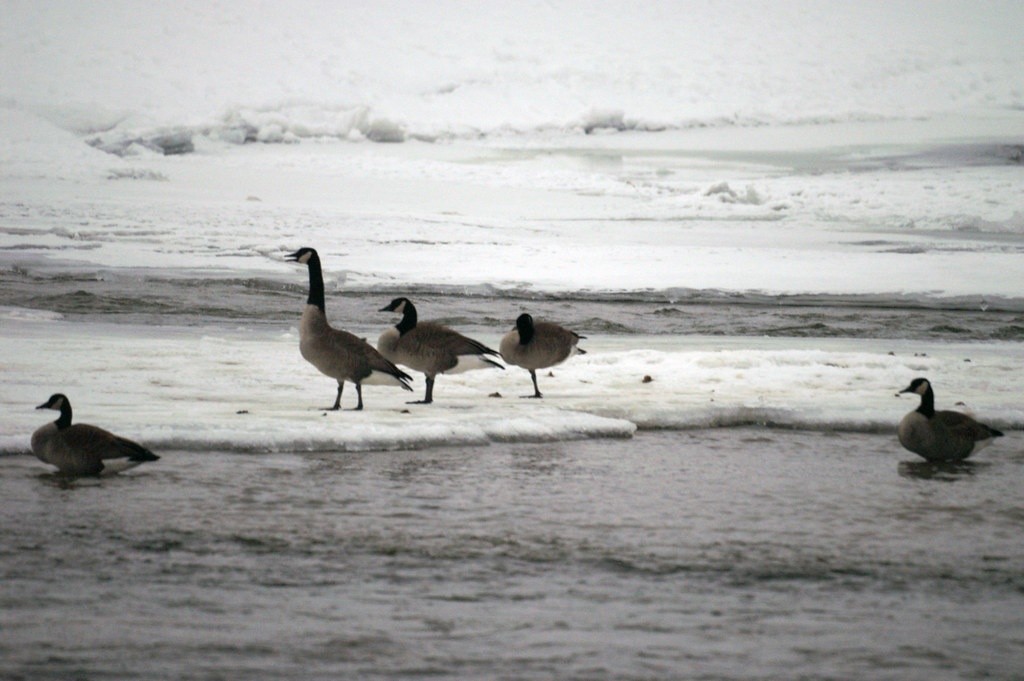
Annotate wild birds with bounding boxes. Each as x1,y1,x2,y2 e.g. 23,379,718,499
282,246,416,411
895,376,1008,464
28,390,162,477
376,296,506,407
500,313,590,399
